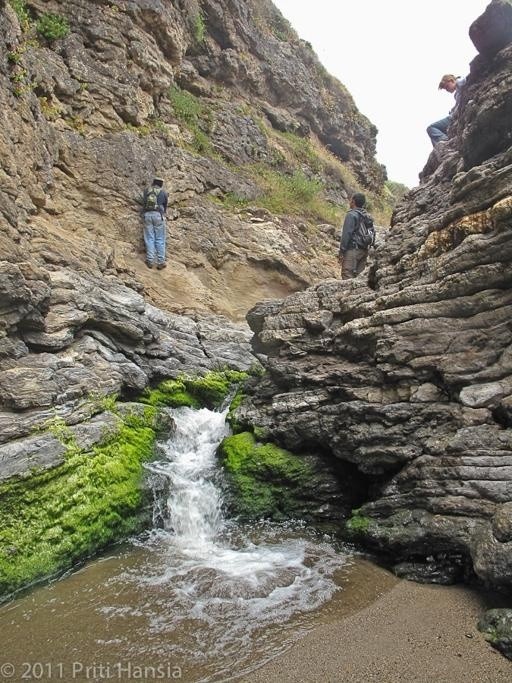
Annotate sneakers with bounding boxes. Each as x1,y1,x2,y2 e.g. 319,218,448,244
144,259,166,269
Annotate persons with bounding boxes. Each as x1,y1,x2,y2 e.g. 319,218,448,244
426,72,467,147
143,177,169,270
338,192,377,280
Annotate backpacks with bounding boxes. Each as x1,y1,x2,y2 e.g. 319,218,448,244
146,187,161,211
354,209,375,250
